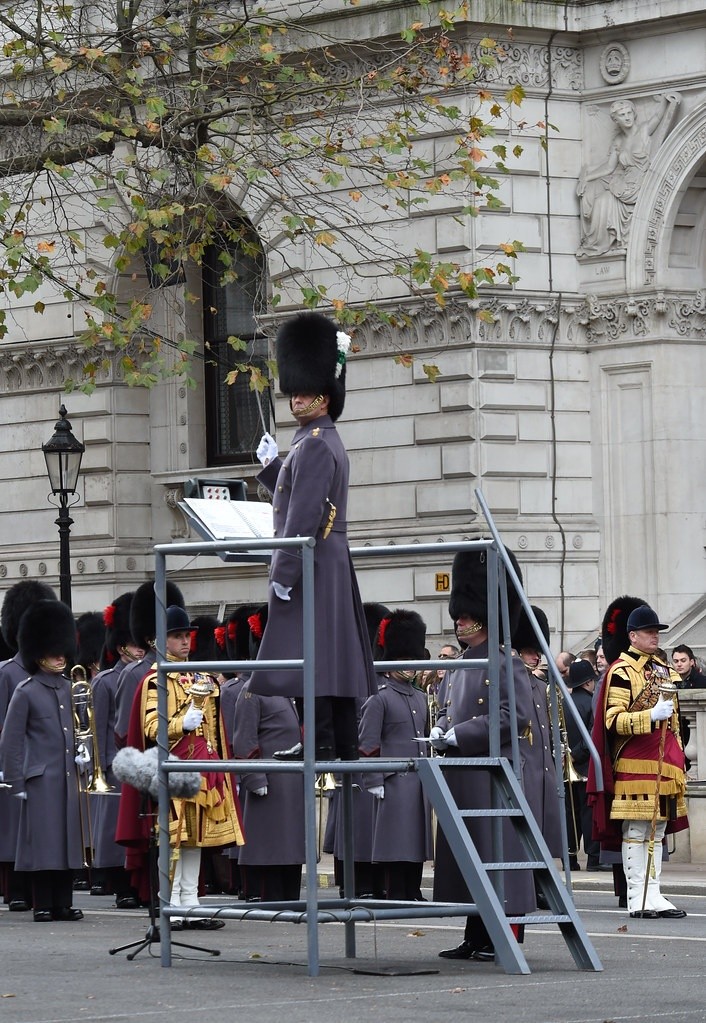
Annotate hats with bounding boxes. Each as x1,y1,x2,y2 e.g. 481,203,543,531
274,312,351,423
0,539,666,688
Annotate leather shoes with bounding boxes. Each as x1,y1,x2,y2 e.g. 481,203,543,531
473,944,495,961
563,863,580,871
273,743,335,762
4,877,429,930
659,909,687,917
335,744,359,760
439,941,480,958
630,910,659,918
587,862,613,871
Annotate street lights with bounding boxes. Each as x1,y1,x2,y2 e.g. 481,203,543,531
41,404,85,679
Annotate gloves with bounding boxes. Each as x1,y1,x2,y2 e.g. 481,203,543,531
252,785,268,796
183,701,203,730
255,432,278,469
0,771,4,782
272,581,292,601
429,727,448,751
651,694,674,722
443,727,459,748
368,785,384,799
74,745,90,767
13,792,27,800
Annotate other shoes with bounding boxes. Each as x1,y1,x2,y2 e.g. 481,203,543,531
619,899,627,907
535,892,551,909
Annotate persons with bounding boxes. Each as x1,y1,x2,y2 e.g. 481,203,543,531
114,580,246,932
586,595,689,919
247,312,378,761
430,535,532,961
0,580,706,912
0,599,90,921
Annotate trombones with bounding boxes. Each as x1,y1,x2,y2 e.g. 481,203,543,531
427,683,445,864
545,683,584,856
68,665,116,870
314,772,337,865
